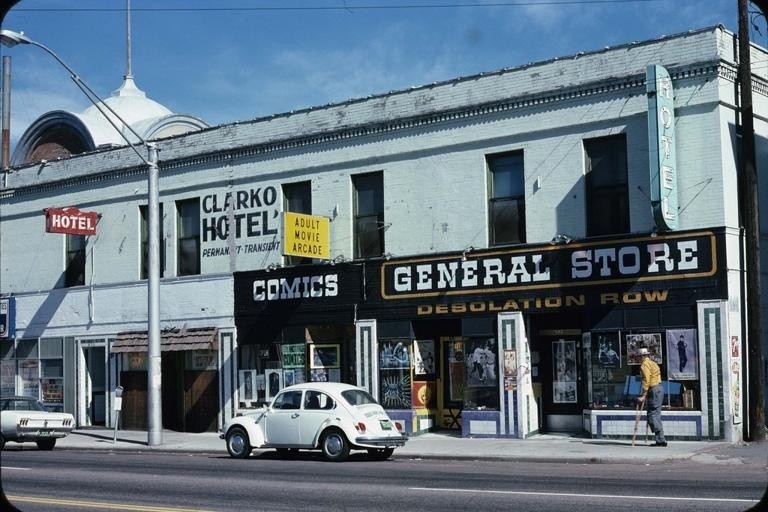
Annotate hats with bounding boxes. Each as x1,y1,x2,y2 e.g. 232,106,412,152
635,348,651,357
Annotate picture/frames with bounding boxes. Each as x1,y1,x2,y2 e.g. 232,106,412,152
309,343,340,369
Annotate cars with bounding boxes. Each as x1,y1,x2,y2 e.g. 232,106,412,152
0,395,77,452
217,382,413,461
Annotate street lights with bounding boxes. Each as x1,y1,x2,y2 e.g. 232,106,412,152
0,27,166,449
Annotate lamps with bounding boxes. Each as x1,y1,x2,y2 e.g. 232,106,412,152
321,254,345,265
383,250,394,259
461,245,475,261
550,232,573,245
263,262,281,273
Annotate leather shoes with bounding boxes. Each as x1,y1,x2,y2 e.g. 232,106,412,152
651,441,667,447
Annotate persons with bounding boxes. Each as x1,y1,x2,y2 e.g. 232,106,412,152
600,341,620,362
469,344,495,382
634,348,667,446
677,335,688,373
